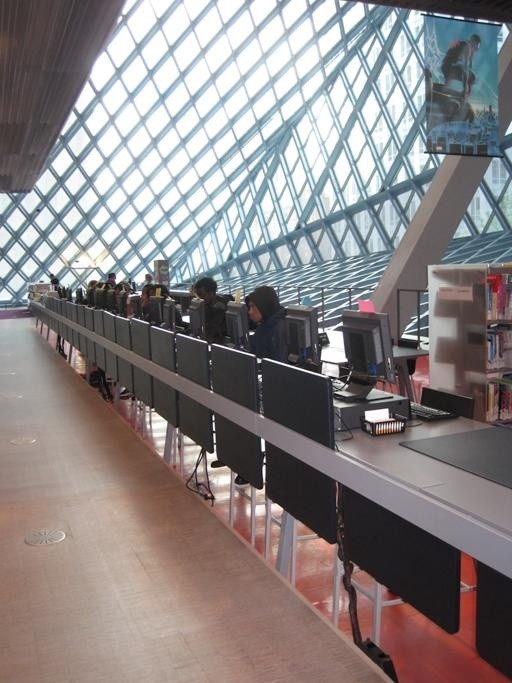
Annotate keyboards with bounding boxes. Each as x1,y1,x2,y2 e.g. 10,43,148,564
408,402,457,420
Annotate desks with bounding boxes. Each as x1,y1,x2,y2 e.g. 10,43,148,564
265,378,512,578
314,327,429,401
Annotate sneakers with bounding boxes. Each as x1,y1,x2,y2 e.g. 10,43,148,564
119,388,131,397
210,460,225,468
234,474,250,485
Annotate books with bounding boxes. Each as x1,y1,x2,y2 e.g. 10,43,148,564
487,273,511,422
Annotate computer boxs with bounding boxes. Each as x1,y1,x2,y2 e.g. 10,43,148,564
333,384,409,432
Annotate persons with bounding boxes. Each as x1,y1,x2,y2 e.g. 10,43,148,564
231,284,287,487
87,272,181,325
440,32,482,102
194,277,236,341
50,273,59,290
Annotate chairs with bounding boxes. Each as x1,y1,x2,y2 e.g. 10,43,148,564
396,337,421,402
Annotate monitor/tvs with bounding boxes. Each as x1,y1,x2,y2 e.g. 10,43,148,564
60,285,319,367
334,308,396,402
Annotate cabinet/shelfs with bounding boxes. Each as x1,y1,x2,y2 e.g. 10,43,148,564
488,320,512,374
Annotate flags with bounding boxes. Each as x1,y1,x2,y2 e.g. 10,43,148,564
422,13,502,158
